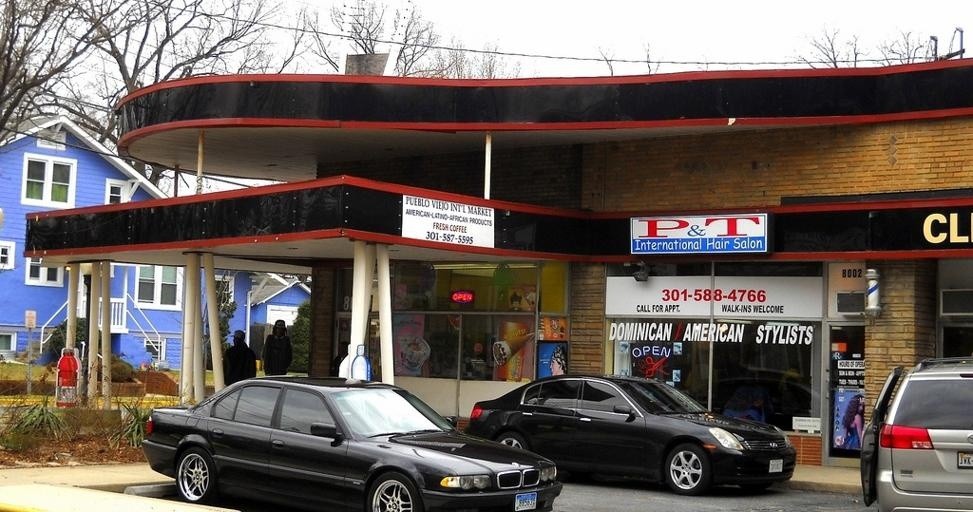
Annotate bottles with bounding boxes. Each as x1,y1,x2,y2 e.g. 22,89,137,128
348,343,372,382
54,348,79,409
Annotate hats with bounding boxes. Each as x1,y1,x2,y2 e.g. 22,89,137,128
234,331,244,339
275,320,285,329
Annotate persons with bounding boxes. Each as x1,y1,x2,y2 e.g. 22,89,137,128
262,320,293,376
224,331,256,386
843,395,865,449
550,346,566,376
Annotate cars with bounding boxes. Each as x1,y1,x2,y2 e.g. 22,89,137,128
143,377,562,512
465,372,798,494
857,354,973,512
